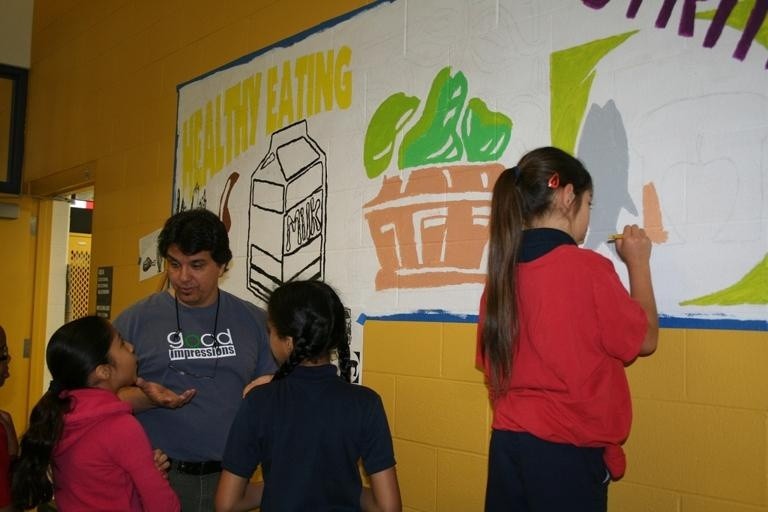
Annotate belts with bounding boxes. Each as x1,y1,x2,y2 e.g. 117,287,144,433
168,457,223,477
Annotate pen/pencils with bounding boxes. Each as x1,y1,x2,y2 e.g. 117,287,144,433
608,234,625,239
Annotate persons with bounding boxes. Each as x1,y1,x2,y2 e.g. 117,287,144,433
213,278,403,512
477,146,659,512
10,314,181,512
0,325,20,511
105,206,280,512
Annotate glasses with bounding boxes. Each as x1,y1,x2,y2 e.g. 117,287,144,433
169,359,219,382
